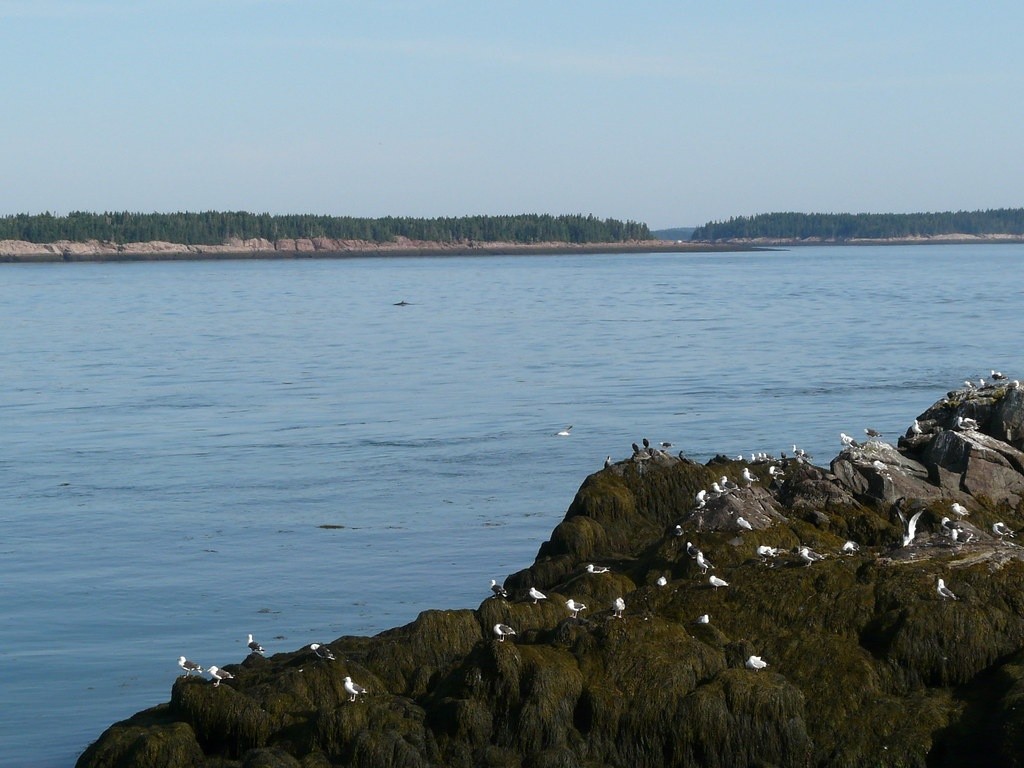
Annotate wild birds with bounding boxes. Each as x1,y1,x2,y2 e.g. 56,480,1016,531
528,587,547,604
491,618,517,642
554,424,573,437
604,370,1019,671
311,644,336,661
585,564,611,573
207,666,234,687
177,655,205,677
490,579,508,600
344,677,368,702
566,599,587,619
247,634,266,655
